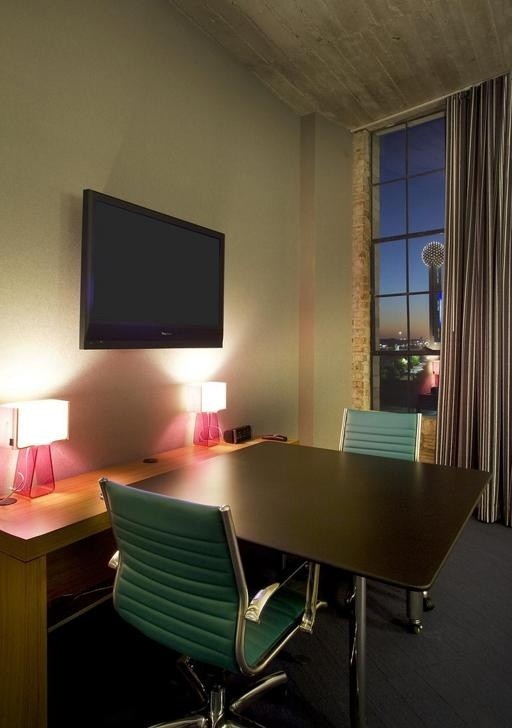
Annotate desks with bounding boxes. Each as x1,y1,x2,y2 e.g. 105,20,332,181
128,440,493,728
0,433,298,728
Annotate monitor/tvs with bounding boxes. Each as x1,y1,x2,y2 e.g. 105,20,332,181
77,188,227,352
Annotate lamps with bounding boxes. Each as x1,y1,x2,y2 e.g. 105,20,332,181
0,398,71,499
186,381,227,446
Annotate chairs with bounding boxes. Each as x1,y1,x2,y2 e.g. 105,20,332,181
322,408,422,635
97,476,323,727
